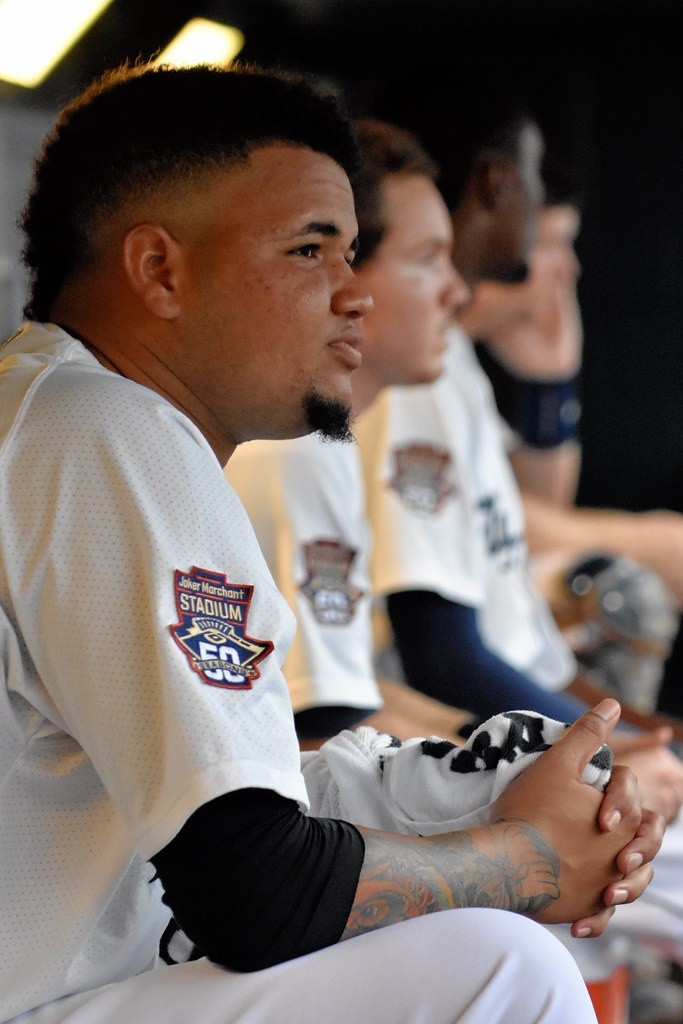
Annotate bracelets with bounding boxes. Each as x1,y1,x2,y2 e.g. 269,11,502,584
477,340,587,450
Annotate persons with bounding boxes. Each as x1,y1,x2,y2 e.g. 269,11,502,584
3,60,683,1023
